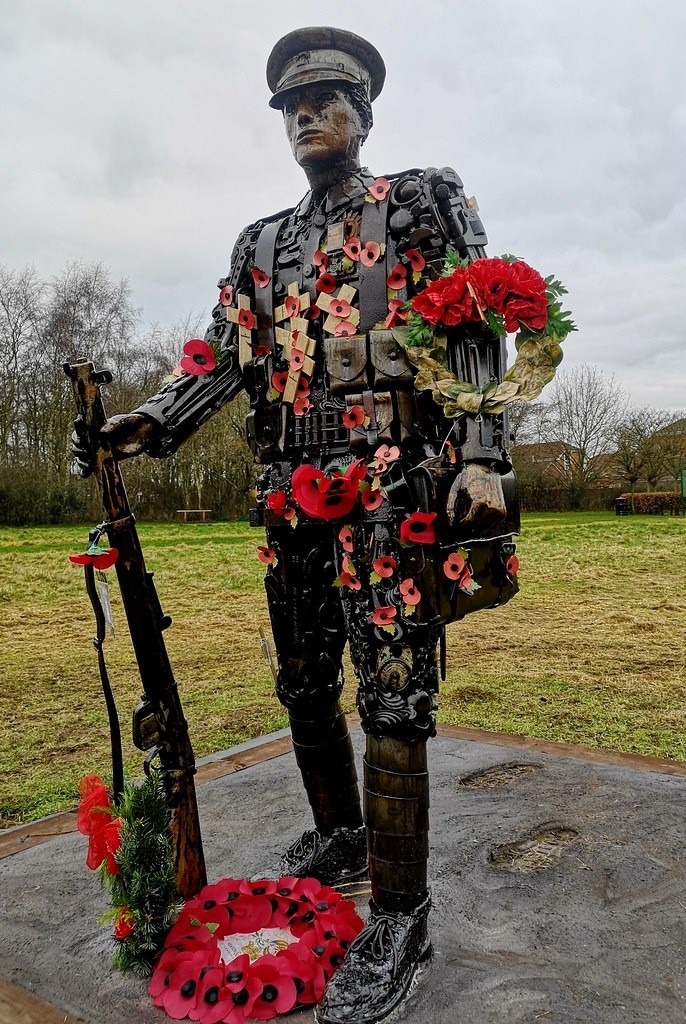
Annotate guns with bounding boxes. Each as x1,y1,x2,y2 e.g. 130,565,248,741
60,356,208,896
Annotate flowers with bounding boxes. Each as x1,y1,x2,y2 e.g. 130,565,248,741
404,249,575,348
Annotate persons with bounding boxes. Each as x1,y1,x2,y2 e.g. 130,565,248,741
71,26,513,1024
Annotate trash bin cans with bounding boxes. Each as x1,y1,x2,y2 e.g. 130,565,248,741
615,497,629,516
